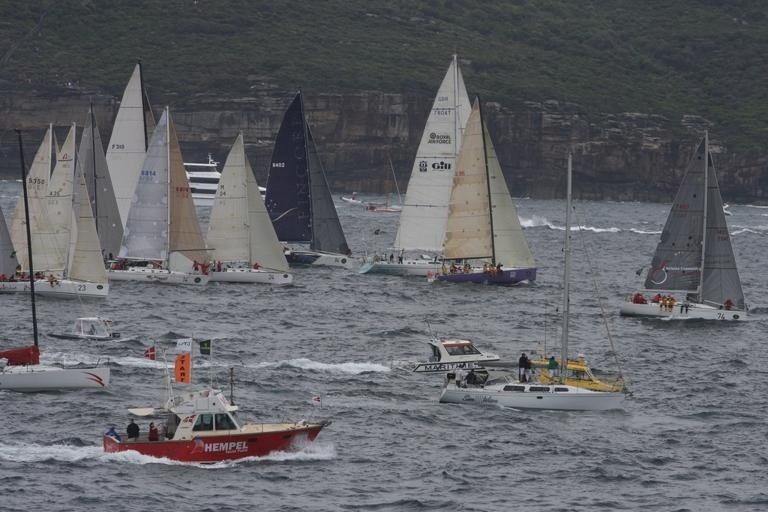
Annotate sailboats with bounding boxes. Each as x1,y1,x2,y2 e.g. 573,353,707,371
617,126,766,326
437,153,633,415
1,130,112,393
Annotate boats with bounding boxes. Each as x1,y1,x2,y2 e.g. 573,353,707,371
534,352,623,394
44,315,123,345
101,334,332,464
411,320,499,373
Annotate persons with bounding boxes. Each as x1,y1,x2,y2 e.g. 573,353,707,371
0,252,59,283
370,249,505,276
519,352,528,382
106,426,121,444
148,421,158,441
453,366,464,388
107,252,262,277
724,298,735,310
631,291,688,314
548,355,559,377
89,323,98,335
127,418,140,441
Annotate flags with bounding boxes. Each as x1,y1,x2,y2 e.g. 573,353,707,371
141,347,155,362
174,339,192,352
199,340,211,356
